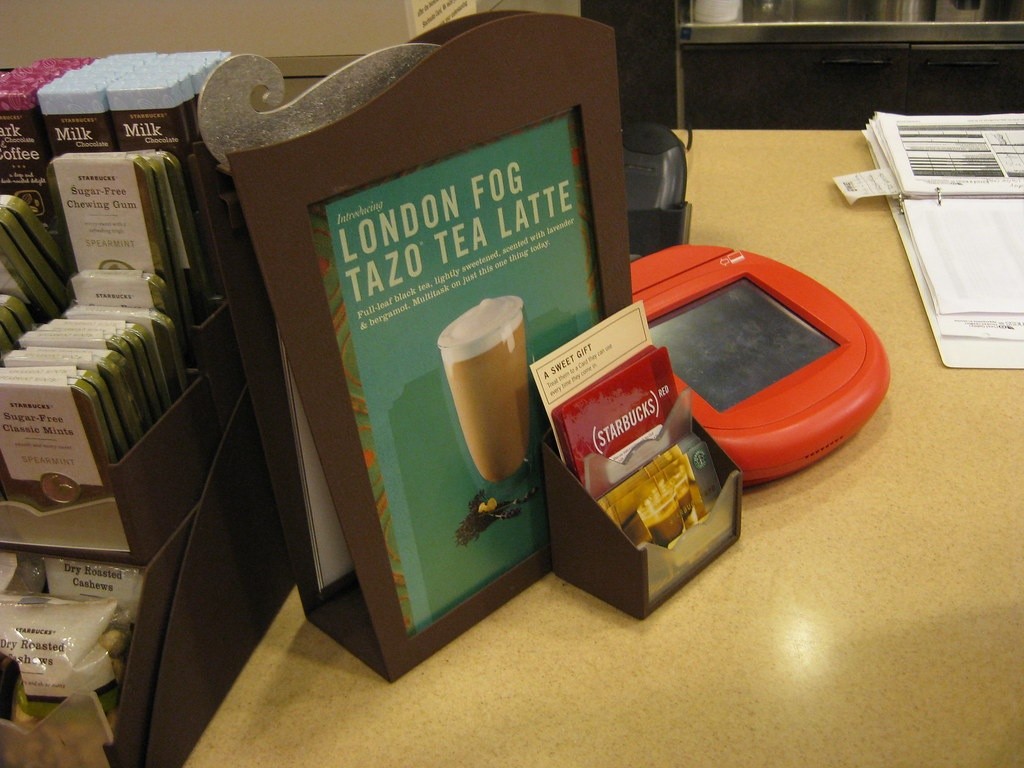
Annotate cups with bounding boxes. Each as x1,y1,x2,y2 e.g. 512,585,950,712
436,294,532,483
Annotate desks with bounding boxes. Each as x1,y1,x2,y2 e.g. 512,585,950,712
180,130,1024,768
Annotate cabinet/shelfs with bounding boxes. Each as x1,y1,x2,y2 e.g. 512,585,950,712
674,40,1024,132
